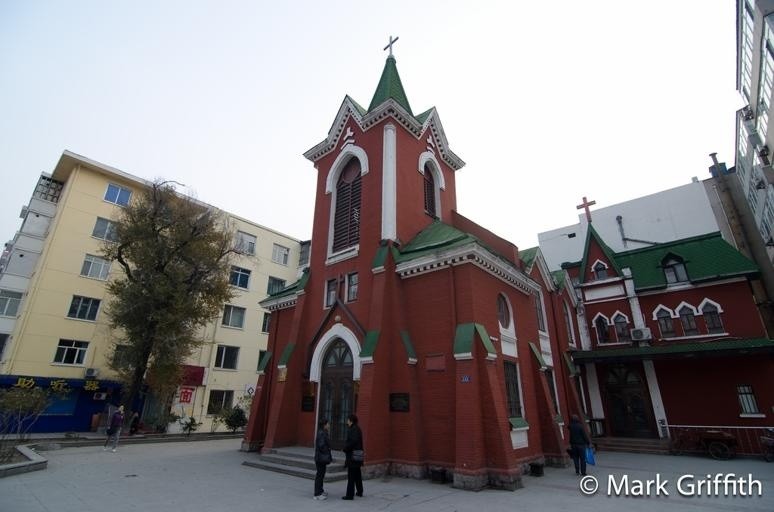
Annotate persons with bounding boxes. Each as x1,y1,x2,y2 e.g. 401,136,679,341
568,413,590,476
312,417,332,501
102,405,124,452
340,413,364,500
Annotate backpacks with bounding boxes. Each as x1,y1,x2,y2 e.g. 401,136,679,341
350,449,364,461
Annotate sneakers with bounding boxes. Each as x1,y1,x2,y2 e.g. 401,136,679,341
313,490,329,501
356,492,363,497
341,495,354,500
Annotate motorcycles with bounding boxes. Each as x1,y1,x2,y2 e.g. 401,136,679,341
759,427,773,462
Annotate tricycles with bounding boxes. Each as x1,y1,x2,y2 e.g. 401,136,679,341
669,427,737,460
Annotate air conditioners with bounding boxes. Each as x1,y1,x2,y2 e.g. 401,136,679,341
92,392,107,400
629,327,652,341
83,367,98,378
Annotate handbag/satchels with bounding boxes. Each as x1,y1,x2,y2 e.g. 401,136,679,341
318,453,331,464
586,447,596,466
